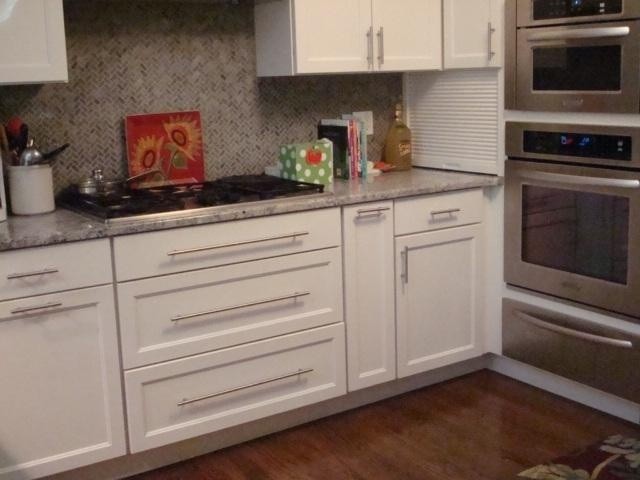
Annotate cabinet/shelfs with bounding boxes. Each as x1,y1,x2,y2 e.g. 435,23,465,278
397,187,492,399
0,238,124,480
344,182,394,412
0,0,71,87
252,0,442,77
444,2,504,71
109,205,345,478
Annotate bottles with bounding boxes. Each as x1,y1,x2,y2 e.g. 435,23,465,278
384,102,412,171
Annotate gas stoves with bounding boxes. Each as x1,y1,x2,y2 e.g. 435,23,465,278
56,174,326,224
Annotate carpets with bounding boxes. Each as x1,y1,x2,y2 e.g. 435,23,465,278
514,432,639,478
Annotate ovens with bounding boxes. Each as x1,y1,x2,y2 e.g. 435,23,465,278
501,120,640,407
504,1,639,115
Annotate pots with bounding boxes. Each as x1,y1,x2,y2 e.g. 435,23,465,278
75,165,162,199
